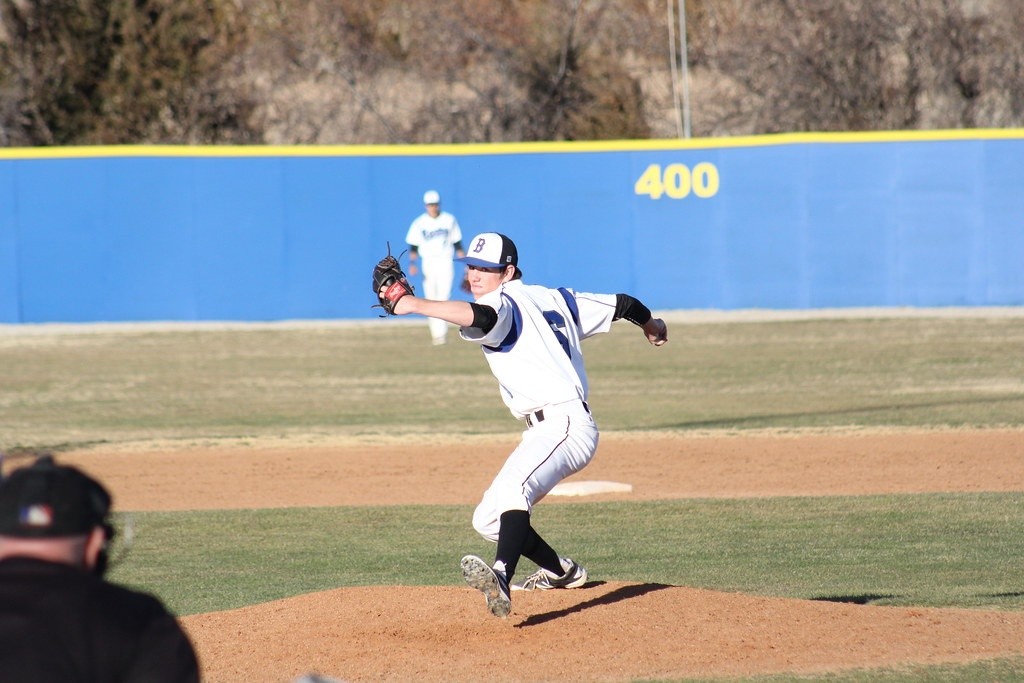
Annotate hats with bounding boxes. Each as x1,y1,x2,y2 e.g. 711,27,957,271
0,456,115,541
453,232,518,268
423,190,440,203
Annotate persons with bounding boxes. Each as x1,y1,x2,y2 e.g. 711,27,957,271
371,232,669,618
405,190,465,345
0,454,201,683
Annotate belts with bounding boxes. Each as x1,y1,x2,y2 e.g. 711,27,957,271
526,401,589,428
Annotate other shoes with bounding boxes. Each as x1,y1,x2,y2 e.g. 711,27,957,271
433,336,446,345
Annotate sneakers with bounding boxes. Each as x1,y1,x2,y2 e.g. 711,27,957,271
510,557,587,590
460,554,511,617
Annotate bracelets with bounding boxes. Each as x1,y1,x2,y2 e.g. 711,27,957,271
410,260,415,265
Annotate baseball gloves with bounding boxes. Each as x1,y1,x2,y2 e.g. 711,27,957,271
461,279,471,293
370,240,416,319
